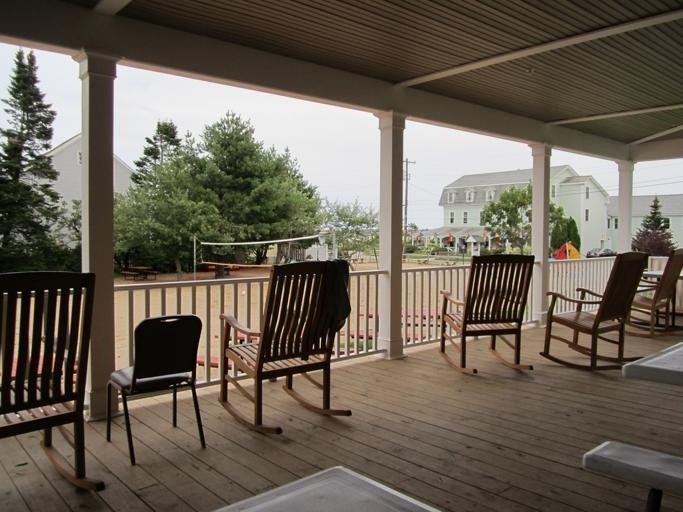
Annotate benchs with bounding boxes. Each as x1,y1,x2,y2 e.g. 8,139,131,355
122,271,138,280
582,441,683,511
144,272,159,278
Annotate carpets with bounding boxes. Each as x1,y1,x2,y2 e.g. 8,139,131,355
213,466,443,512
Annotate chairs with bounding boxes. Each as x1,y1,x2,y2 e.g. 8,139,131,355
439,254,534,375
217,259,352,434
538,253,645,371
1,272,106,491
626,248,683,335
107,314,205,466
